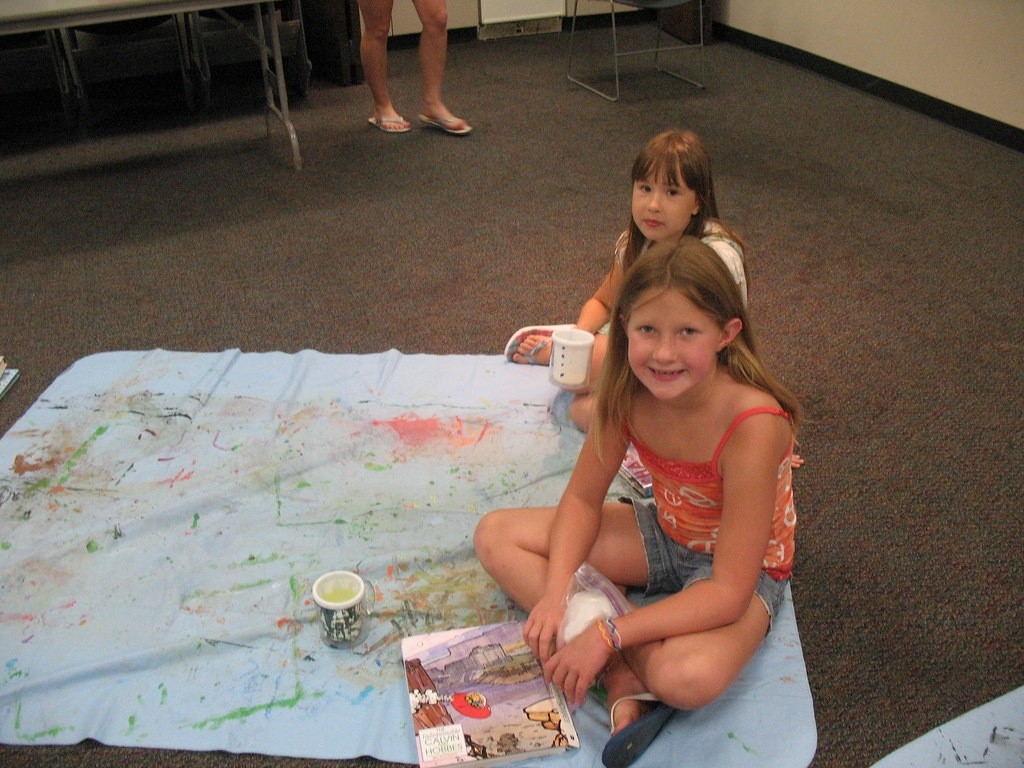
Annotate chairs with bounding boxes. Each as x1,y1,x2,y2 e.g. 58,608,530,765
565,0,709,102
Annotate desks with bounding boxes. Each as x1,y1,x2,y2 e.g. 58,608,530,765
0,1,304,174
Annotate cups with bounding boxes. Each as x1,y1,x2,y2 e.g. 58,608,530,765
311,571,374,649
547,327,595,389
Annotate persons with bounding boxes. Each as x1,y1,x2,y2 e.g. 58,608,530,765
357,0,471,134
473,236,795,768
503,130,748,432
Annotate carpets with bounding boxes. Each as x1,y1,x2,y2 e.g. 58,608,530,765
868,685,1024,768
0,347,819,767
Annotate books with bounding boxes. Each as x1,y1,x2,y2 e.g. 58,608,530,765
400,620,580,768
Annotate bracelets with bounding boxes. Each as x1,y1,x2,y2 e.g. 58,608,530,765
596,618,622,653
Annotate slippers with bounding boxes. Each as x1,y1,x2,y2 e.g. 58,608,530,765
418,113,473,135
504,324,578,367
602,694,673,768
368,115,411,133
